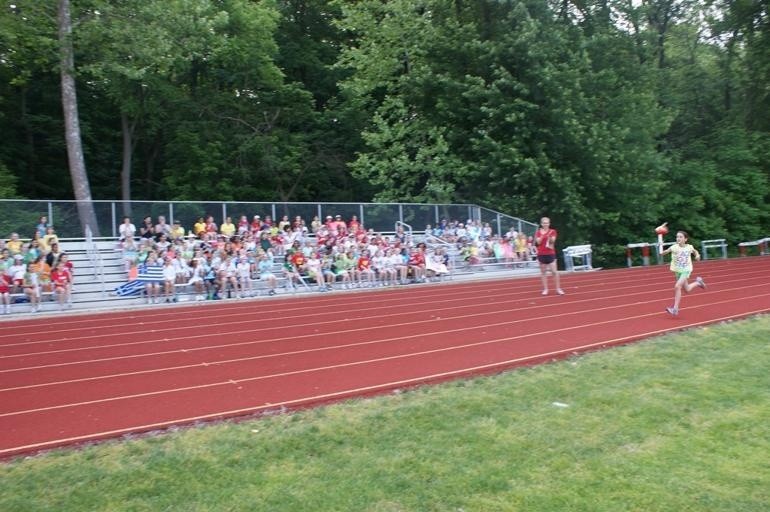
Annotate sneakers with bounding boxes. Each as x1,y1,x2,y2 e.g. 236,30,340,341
196,291,256,301
0,299,74,315
666,308,678,315
318,274,429,292
147,296,177,304
697,277,706,289
268,290,277,295
542,289,565,294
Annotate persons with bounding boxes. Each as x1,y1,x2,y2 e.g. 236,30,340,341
426,218,539,270
658,231,707,316
0,215,73,313
119,215,448,304
535,218,565,296
